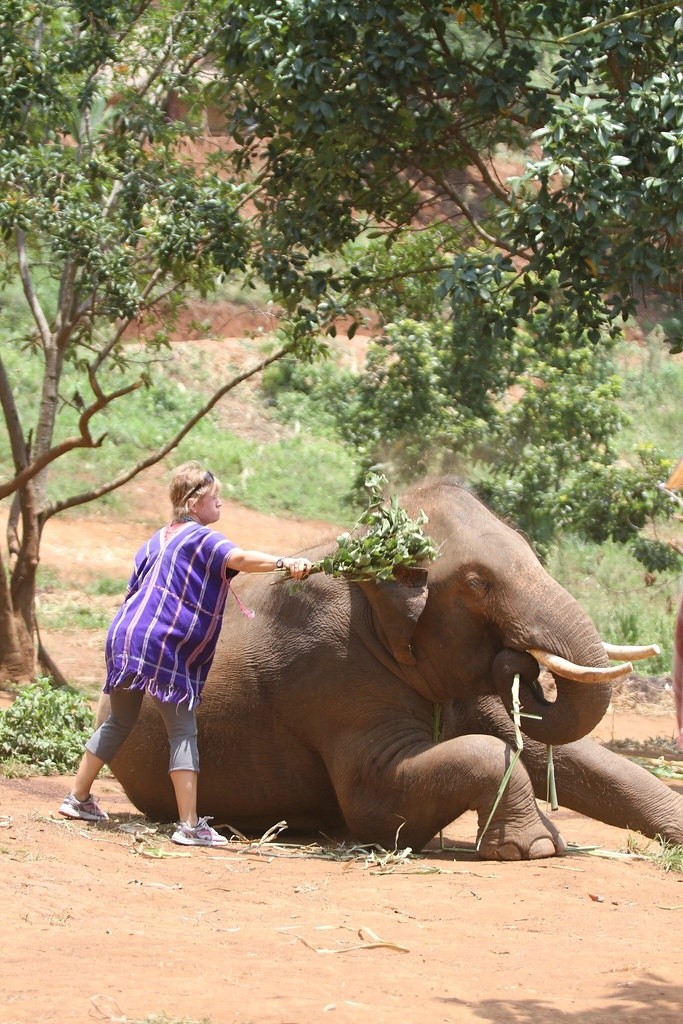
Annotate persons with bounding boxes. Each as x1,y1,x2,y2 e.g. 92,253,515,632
59,461,310,846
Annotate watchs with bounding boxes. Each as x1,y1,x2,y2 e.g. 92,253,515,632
276,558,284,568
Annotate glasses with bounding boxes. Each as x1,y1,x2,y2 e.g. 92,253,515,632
183,470,215,504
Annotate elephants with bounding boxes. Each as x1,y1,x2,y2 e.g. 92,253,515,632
99,478,681,860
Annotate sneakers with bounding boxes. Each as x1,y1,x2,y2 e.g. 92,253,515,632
171,816,228,846
58,793,110,821
